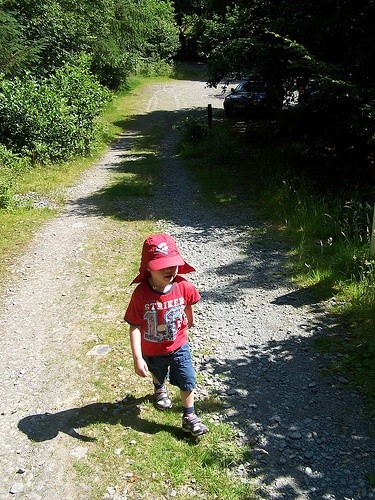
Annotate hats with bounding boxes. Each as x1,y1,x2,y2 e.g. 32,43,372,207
131,235,197,285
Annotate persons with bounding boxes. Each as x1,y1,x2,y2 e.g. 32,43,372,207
124,234,211,437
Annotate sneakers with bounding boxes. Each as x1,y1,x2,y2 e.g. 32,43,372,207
154,387,172,410
183,414,209,436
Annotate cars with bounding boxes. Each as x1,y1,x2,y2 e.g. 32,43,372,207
223,80,269,116
